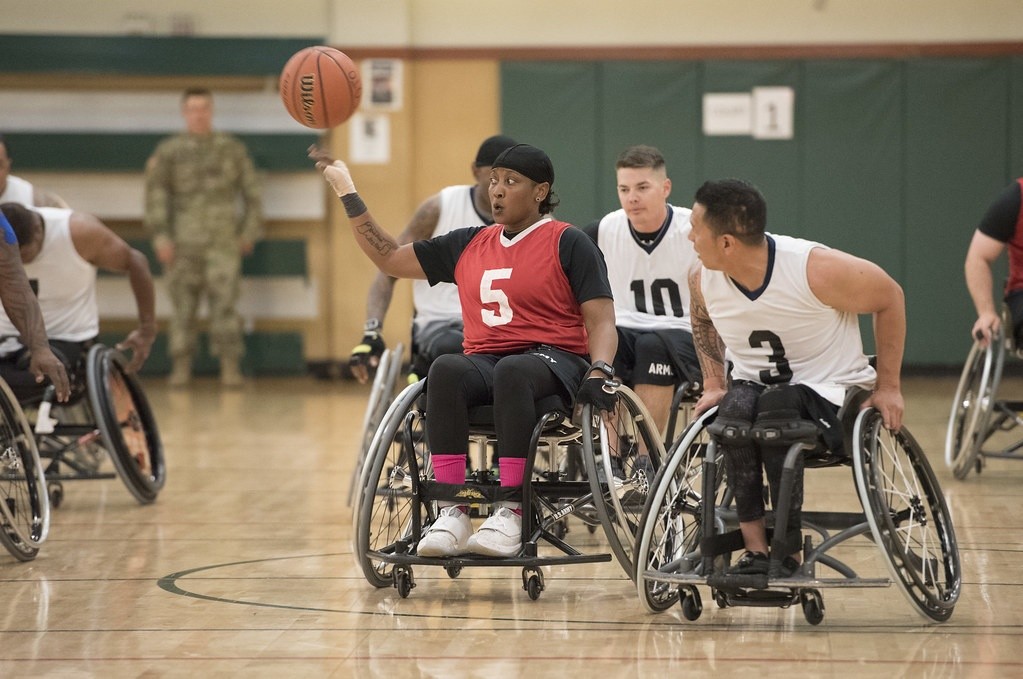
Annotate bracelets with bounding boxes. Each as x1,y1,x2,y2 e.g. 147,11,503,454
340,193,367,218
364,318,383,331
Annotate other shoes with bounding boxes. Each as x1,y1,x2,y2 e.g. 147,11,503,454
166,357,193,388
589,455,655,506
218,353,243,390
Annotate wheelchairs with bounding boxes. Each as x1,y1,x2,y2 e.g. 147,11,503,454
944,300,1023,479
633,354,962,624
344,343,704,539
352,376,684,600
0,377,50,562
0,343,166,504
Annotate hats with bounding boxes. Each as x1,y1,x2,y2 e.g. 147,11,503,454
492,143,555,187
474,134,518,165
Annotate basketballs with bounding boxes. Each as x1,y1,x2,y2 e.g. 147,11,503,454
279,45,364,129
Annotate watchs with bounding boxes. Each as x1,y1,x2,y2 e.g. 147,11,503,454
582,360,615,381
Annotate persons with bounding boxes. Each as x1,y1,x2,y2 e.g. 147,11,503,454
579,144,735,510
143,84,269,392
963,176,1023,363
0,140,158,416
305,134,620,560
686,178,907,599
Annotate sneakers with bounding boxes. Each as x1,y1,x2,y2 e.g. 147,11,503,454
468,506,523,558
417,503,474,558
717,550,799,600
